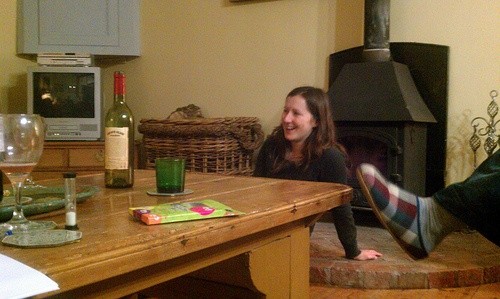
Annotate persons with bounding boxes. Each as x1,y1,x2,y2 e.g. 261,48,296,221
254,85,383,260
356,148,500,260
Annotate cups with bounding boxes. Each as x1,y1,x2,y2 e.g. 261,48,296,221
155,157,187,193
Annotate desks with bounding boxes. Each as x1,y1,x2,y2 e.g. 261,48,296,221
0,168,354,299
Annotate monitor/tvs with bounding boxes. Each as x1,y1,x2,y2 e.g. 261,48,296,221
27,65,102,141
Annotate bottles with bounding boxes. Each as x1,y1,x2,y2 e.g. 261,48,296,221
105,70,134,189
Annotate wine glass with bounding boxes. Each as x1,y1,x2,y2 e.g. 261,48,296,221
0,113,57,235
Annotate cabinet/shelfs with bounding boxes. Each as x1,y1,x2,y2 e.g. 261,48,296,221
28,141,141,180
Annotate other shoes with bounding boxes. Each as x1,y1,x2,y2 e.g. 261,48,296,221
356,163,429,261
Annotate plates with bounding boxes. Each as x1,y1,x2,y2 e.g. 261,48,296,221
0,183,101,223
